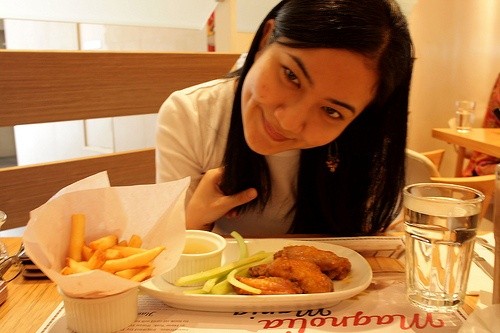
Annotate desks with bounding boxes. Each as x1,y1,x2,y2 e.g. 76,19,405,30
432,128,500,177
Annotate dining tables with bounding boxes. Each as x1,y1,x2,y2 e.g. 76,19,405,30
0,227,500,333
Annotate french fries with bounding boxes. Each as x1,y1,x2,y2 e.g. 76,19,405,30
59,215,167,283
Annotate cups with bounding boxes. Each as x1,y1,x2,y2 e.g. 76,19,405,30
400,183,485,314
456,100,475,133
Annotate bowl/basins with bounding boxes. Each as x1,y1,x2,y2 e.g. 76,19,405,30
57,283,137,333
160,230,226,284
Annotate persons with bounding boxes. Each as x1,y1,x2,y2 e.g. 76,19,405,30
470,73,500,176
155,0,417,235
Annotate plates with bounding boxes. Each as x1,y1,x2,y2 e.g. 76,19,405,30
140,238,372,312
